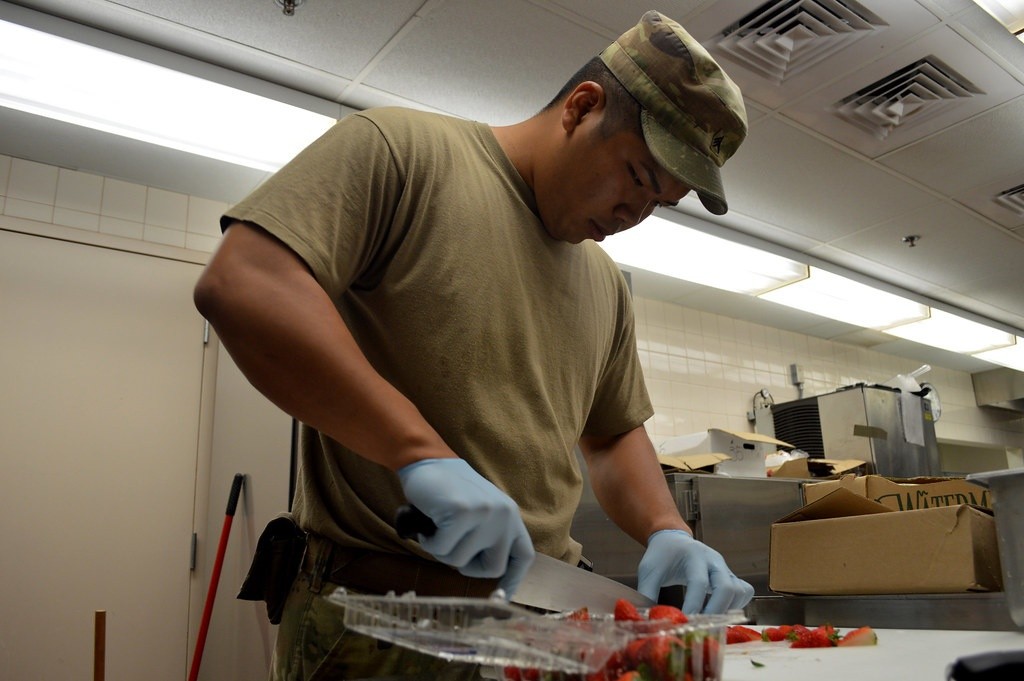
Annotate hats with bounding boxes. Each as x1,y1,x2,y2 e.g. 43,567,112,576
599,9,748,215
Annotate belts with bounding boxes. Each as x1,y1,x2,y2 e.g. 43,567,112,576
302,536,502,595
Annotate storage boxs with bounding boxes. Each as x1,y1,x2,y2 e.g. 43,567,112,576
660,426,796,477
799,473,992,511
320,585,753,681
768,487,1003,595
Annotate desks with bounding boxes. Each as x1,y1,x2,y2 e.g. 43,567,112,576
721,625,1024,681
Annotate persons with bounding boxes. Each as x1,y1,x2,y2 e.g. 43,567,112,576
193,10,755,681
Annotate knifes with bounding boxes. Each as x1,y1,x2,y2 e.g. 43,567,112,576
395,505,660,620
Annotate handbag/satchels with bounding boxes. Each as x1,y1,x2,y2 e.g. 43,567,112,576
236,517,307,624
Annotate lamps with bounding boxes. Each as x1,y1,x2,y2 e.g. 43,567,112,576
0,0,1024,374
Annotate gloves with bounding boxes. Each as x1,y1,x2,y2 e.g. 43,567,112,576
638,530,755,615
398,457,535,601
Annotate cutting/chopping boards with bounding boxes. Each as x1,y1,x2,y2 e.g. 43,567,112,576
479,624,1024,681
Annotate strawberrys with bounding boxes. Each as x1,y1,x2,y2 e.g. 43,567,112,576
501,599,721,681
723,622,878,648
